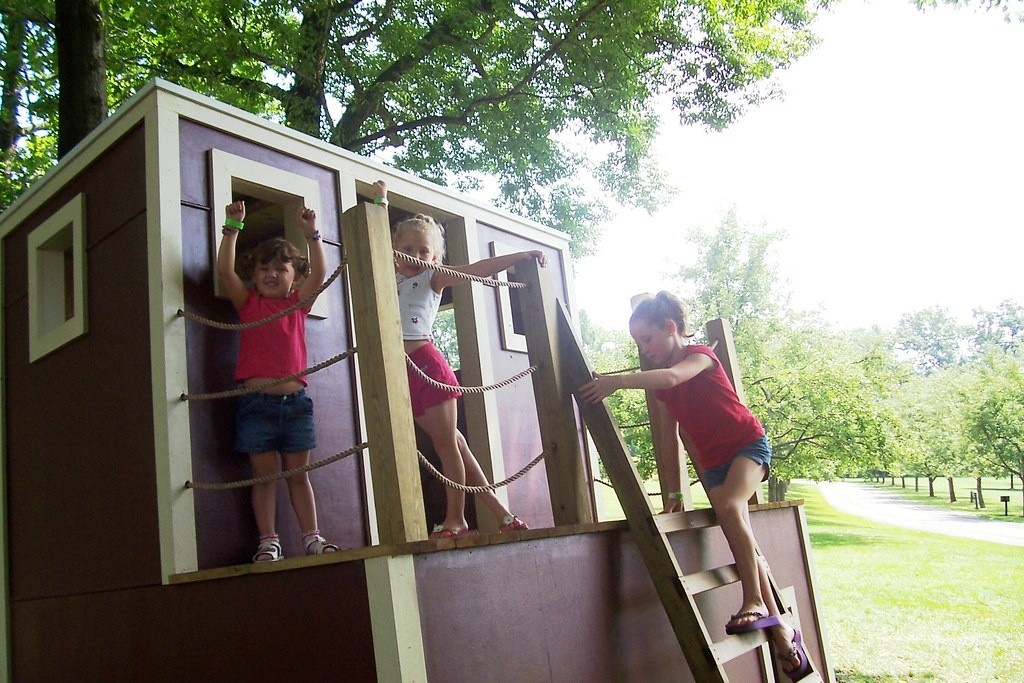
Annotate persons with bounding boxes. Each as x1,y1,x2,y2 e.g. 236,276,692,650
218,202,339,563
580,290,808,679
372,180,548,537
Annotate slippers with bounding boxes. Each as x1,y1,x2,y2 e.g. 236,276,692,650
777,628,808,678
429,524,479,540
500,515,528,532
726,612,779,635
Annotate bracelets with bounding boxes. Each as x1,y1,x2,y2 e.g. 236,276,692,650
306,229,320,241
668,492,683,499
222,226,238,235
225,219,244,229
373,198,390,204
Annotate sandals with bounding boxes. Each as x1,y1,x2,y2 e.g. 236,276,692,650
304,536,340,558
253,540,281,563
